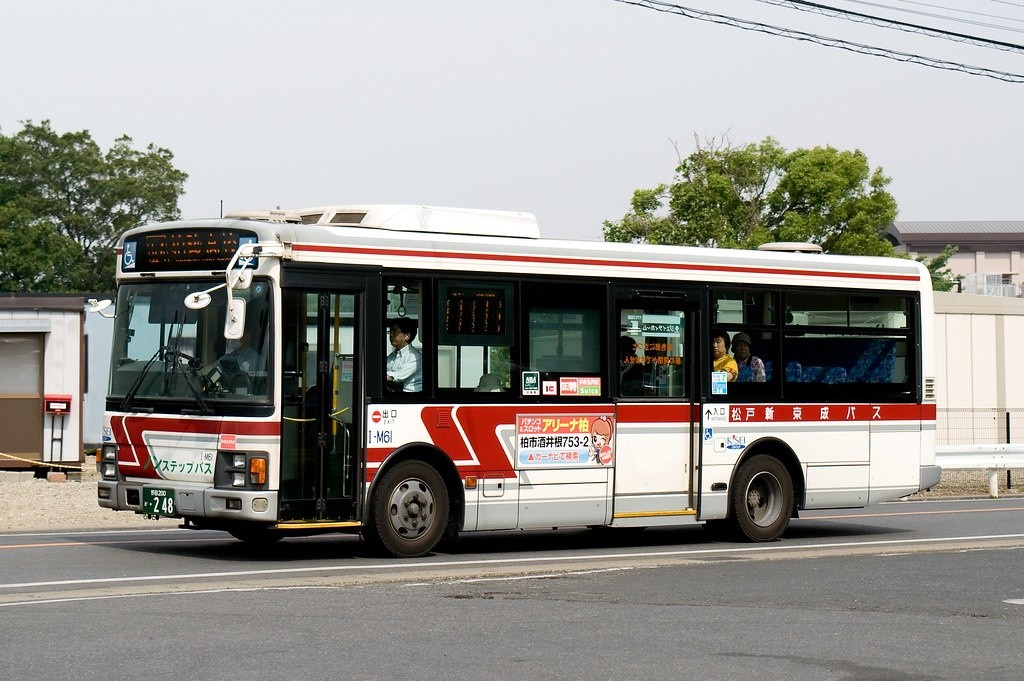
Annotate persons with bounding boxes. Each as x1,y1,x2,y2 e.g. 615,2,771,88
714,332,739,381
619,336,645,396
386,317,423,392
206,327,260,371
731,333,765,382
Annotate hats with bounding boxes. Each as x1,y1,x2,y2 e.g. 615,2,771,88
731,333,752,350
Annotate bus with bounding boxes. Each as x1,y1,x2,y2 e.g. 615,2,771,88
88,206,942,559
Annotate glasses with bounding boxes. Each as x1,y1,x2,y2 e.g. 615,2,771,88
386,330,403,334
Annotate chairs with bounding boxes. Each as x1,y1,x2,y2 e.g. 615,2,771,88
473,338,900,392
